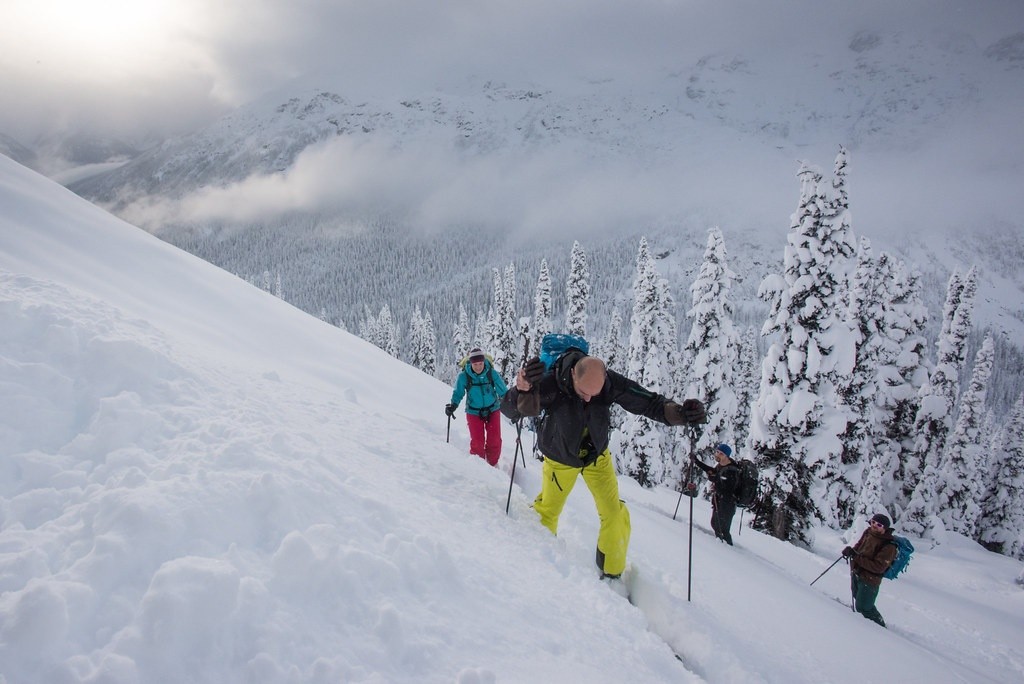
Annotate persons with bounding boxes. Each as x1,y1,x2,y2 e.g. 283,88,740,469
689,444,740,546
842,514,898,627
445,347,508,468
502,347,707,580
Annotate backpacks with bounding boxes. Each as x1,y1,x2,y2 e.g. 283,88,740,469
718,459,758,512
537,335,591,375
883,535,914,581
456,351,494,392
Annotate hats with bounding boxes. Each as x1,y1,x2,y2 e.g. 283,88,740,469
469,347,485,364
716,444,732,457
872,514,890,528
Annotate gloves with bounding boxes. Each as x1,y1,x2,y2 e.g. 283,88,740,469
664,399,708,426
445,404,457,420
511,356,547,404
842,546,856,564
